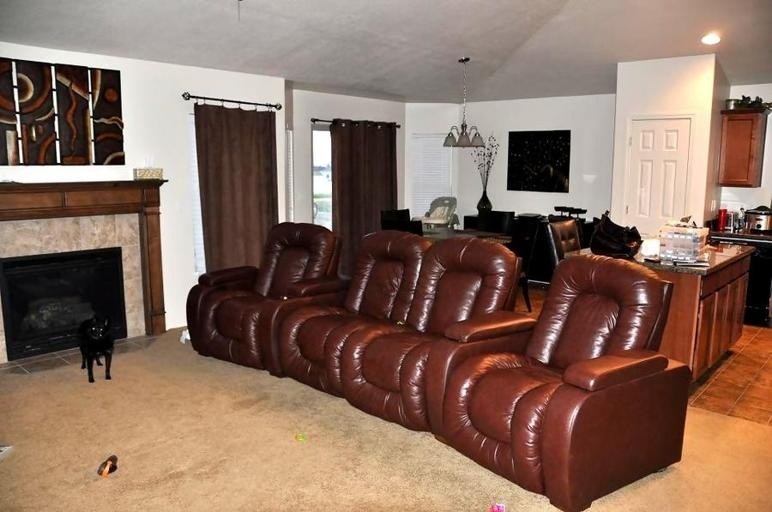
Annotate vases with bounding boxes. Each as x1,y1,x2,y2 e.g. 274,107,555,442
476,187,493,215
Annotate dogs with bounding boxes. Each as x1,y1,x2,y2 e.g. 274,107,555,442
76,312,115,383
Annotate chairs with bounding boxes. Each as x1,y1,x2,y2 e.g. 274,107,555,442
378,208,581,312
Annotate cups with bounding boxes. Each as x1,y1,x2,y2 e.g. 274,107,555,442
717,209,726,232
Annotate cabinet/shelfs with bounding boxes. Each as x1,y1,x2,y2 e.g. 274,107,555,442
653,254,749,382
720,113,766,188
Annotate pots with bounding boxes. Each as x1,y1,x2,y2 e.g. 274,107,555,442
727,97,763,113
746,206,772,234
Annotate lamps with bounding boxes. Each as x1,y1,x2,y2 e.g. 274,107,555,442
441,58,483,148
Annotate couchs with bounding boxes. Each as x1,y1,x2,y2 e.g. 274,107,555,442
424,253,690,511
281,232,434,394
340,238,523,432
186,221,339,370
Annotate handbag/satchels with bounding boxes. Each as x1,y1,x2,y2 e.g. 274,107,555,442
590,209,644,261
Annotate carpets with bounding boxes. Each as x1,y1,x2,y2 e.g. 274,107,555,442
1,325,770,510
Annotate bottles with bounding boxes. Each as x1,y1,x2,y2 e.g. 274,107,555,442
738,208,745,228
